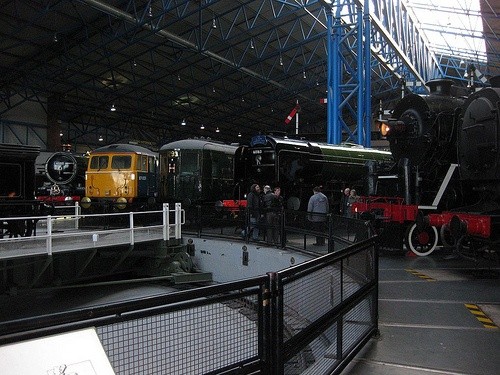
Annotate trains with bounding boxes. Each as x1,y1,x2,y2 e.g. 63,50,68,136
79,143,160,218
230,133,393,217
347,77,500,272
151,138,239,225
0,139,88,238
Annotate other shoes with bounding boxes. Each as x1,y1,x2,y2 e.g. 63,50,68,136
313,243,319,246
248,238,254,242
241,230,245,236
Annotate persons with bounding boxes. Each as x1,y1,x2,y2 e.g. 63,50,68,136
308,187,329,246
241,184,283,243
340,188,357,235
